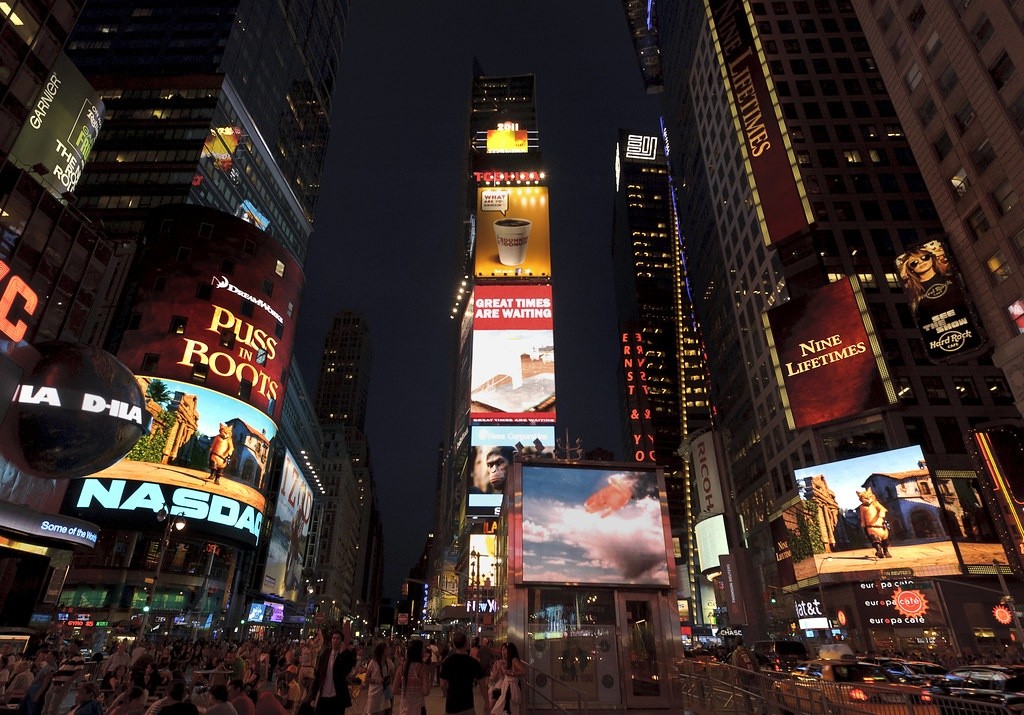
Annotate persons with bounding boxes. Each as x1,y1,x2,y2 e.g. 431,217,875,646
439,631,489,715
228,679,256,715
488,643,508,711
469,636,496,701
247,689,290,715
365,642,395,715
106,675,149,712
277,665,300,714
17,649,57,715
392,639,429,715
202,684,239,715
63,683,102,715
108,684,144,714
304,631,353,715
158,682,199,715
491,642,529,715
0,650,47,703
702,640,1024,670
101,637,325,690
40,638,84,715
730,635,768,715
143,689,170,715
345,635,453,698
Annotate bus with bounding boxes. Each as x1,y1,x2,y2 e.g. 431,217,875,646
274,616,310,642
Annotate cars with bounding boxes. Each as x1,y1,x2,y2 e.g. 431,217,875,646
767,645,1023,715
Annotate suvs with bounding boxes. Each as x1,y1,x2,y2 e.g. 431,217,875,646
681,647,721,674
746,640,808,675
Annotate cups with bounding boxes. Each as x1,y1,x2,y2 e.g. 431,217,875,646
493,217,532,266
